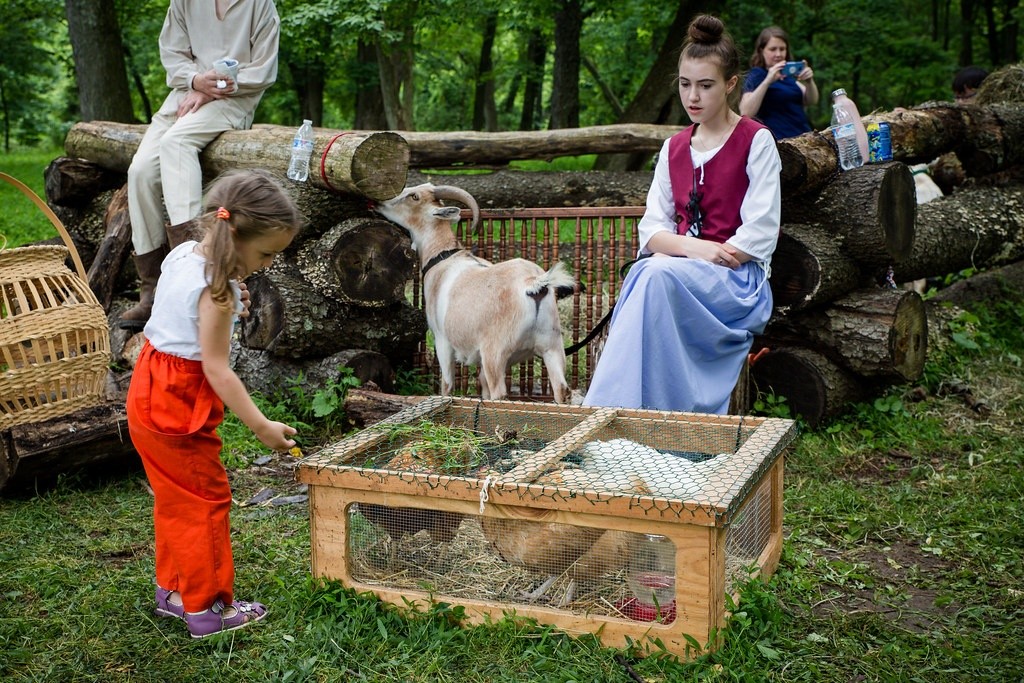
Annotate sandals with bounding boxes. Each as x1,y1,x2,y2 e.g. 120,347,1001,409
186,596,269,640
154,583,188,624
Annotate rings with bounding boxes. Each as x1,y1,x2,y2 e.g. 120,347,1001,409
718,258,723,265
216,79,227,90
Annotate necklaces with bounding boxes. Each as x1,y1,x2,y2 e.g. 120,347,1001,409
697,111,736,150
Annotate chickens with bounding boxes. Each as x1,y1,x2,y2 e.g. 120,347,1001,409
358,435,735,608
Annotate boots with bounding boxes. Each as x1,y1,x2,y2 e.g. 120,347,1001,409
119,241,171,330
165,211,205,252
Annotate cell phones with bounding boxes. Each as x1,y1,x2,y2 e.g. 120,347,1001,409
780,62,804,75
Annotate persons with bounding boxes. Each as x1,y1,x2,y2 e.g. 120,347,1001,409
581,13,988,414
124,167,303,638
119,0,280,330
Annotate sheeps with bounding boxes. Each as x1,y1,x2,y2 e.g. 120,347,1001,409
377,181,580,401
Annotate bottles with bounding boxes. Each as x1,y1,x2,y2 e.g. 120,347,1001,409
832,88,869,164
832,102,864,170
287,120,314,182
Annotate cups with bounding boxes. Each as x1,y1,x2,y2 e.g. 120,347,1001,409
214,59,238,93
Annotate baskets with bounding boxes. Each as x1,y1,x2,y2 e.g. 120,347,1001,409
0,168,111,433
